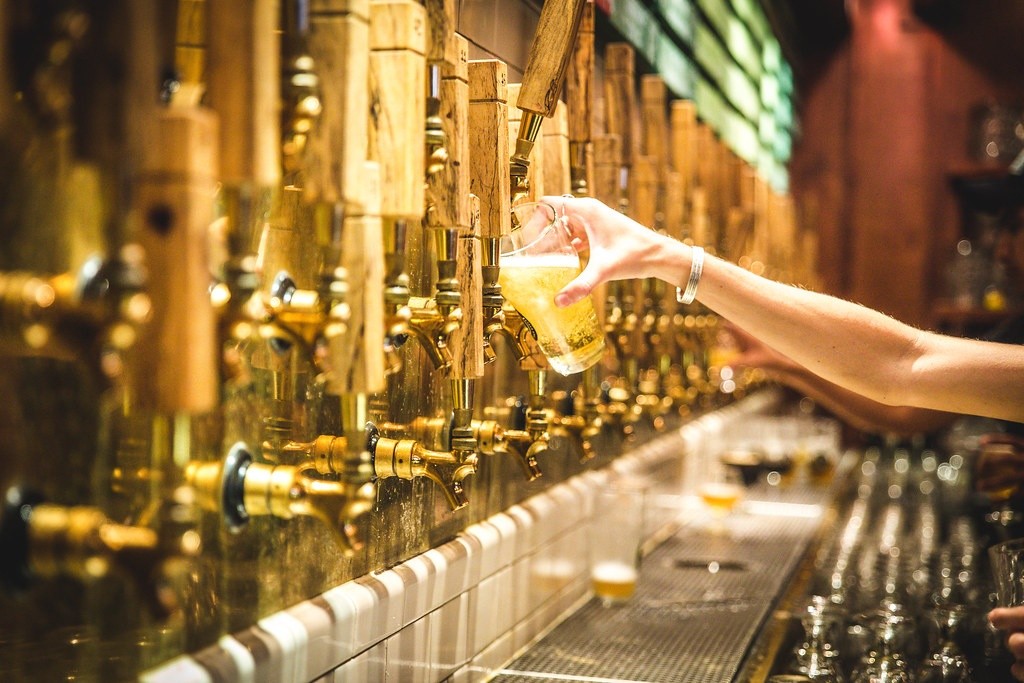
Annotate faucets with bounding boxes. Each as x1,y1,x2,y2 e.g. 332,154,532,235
1,256,779,638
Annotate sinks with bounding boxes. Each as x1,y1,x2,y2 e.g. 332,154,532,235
140,383,856,683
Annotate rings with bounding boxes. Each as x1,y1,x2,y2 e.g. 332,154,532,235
562,194,575,205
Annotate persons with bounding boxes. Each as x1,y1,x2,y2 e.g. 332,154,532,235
723,321,1024,498
520,193,1024,683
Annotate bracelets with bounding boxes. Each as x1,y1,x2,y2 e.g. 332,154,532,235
676,246,704,304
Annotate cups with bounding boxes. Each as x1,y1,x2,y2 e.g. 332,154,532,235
990,538,1024,609
496,202,609,376
700,468,739,515
590,488,644,601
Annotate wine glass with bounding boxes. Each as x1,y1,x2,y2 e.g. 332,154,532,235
774,594,987,683
979,465,1024,523
